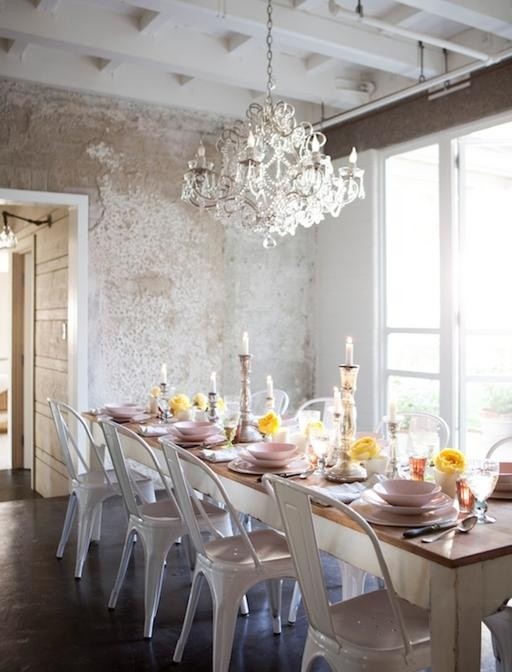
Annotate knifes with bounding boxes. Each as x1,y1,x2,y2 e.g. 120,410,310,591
256,472,301,483
402,518,458,538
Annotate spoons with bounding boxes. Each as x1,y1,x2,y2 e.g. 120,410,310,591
286,472,310,482
421,516,478,543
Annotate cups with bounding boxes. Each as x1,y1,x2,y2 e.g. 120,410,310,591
456,478,474,514
224,395,240,418
408,455,427,480
298,410,320,441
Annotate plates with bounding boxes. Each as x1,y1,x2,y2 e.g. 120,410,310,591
347,498,459,527
157,435,226,447
227,457,308,474
98,414,152,422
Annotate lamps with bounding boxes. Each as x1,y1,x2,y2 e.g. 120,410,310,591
181,0,365,250
0,211,51,252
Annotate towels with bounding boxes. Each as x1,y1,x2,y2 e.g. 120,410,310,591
197,446,241,463
305,482,371,506
138,424,170,436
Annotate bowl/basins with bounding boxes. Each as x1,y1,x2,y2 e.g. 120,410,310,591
247,442,298,460
362,488,452,515
105,403,137,414
174,421,212,436
168,427,220,442
238,449,304,468
101,409,146,419
372,478,442,507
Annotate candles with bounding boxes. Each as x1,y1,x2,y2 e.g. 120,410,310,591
345,336,354,365
240,331,249,353
209,370,218,393
265,375,274,398
332,385,343,410
347,146,357,173
311,133,322,164
387,399,397,418
159,362,168,383
196,140,206,170
246,130,256,162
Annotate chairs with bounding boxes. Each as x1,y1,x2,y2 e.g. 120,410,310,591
158,438,299,671
485,435,512,458
289,410,447,625
296,397,357,430
262,473,435,672
97,418,250,641
47,398,158,580
246,389,289,412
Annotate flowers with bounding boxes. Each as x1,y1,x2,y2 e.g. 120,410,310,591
192,393,208,412
152,386,160,399
348,437,382,460
167,395,190,416
257,414,280,435
217,399,226,410
432,447,465,475
304,420,326,433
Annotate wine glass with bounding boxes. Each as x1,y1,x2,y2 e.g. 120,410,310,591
311,428,335,476
158,387,174,424
463,457,501,524
